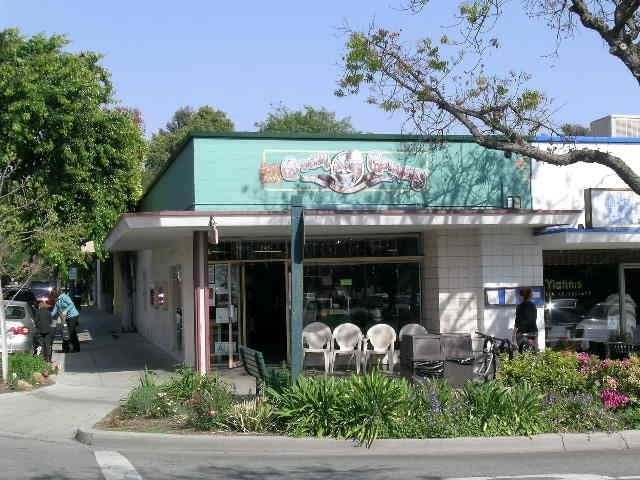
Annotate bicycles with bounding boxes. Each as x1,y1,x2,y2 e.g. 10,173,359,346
474,330,519,380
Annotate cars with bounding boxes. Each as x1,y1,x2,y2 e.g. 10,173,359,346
0,282,86,356
544,297,639,350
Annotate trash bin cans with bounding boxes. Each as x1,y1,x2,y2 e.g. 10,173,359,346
608,342,640,362
400,332,473,389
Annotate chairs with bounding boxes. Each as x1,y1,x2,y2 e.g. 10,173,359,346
301,322,428,375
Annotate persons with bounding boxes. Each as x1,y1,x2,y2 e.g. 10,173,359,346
50,287,81,353
35,302,53,363
512,287,539,352
